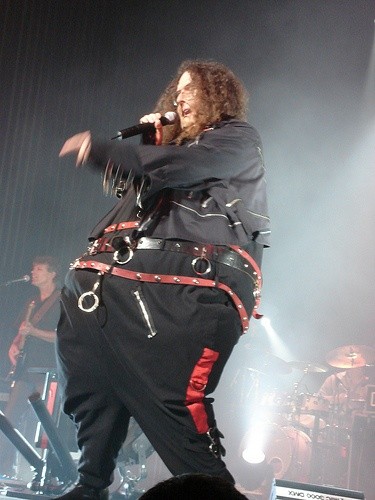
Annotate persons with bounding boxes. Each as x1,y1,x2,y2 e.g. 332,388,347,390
51,59,271,500
8,256,62,451
313,369,368,491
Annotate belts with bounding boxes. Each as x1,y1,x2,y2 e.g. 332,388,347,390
89,237,258,284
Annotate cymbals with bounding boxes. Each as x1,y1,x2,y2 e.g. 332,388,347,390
266,352,288,368
282,355,323,374
326,344,371,367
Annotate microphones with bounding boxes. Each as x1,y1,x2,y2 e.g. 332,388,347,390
112,111,177,140
5,275,30,286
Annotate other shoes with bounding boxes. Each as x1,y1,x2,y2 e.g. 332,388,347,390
53,482,109,500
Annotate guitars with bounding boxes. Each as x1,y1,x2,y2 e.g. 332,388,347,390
12,299,37,368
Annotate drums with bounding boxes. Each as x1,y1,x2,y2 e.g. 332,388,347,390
250,386,371,440
257,423,307,482
296,394,328,427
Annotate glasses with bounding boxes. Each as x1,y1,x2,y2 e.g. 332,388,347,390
172,87,193,106
